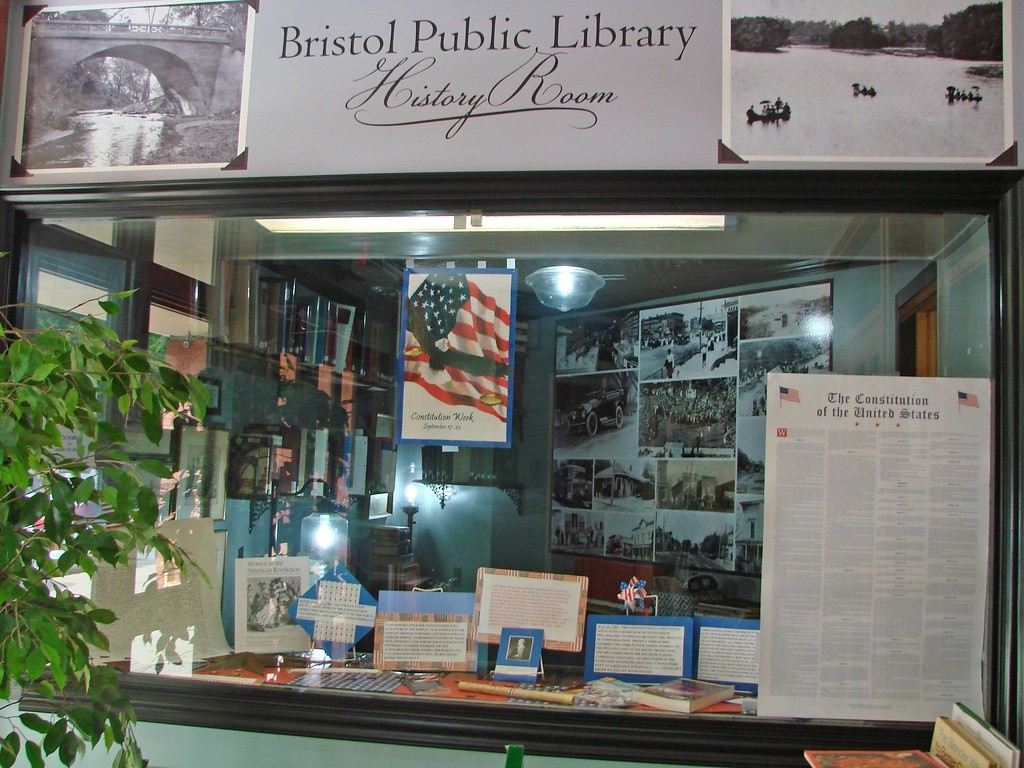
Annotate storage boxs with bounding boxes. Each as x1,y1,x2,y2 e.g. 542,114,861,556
650,590,724,616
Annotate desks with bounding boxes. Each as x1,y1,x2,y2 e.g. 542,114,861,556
94,649,756,715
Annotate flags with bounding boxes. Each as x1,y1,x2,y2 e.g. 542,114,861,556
958,392,980,408
403,273,508,423
780,386,800,404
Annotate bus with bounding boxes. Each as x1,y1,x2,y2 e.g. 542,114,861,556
553,464,593,508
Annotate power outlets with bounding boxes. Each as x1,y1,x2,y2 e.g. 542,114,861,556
453,567,462,587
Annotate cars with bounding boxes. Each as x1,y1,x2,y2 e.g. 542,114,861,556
556,388,628,436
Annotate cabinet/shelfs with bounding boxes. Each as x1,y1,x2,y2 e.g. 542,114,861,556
209,344,395,534
412,352,527,517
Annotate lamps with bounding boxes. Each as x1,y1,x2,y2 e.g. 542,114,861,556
401,485,420,553
267,476,349,571
255,214,741,232
523,259,603,314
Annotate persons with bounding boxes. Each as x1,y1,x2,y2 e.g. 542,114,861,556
691,433,702,455
700,344,708,368
664,348,677,378
748,96,791,116
640,334,690,349
555,521,604,547
509,638,530,660
950,89,979,98
862,86,875,92
